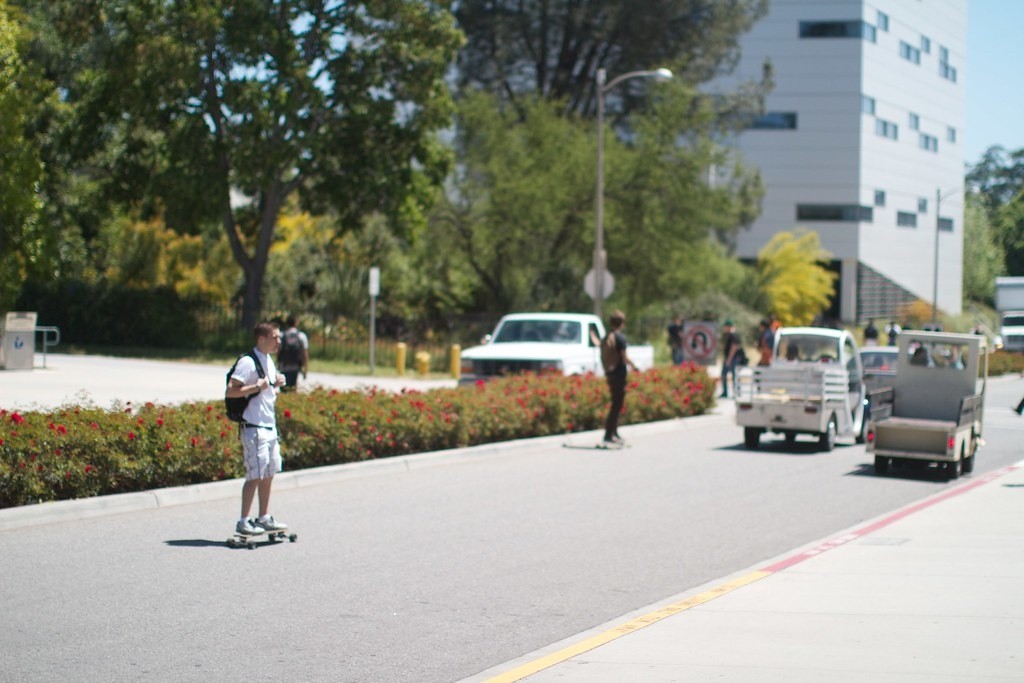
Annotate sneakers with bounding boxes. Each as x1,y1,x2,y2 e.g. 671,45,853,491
256,516,287,530
236,520,265,534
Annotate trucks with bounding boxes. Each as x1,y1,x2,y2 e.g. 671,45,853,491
1000,310,1024,351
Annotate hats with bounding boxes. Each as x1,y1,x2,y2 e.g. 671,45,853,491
725,319,733,325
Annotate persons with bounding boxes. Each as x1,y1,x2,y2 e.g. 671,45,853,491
667,314,683,364
276,315,309,393
589,328,599,346
225,321,288,535
885,320,901,346
755,312,799,365
864,317,879,347
599,311,640,445
912,345,966,371
718,319,742,398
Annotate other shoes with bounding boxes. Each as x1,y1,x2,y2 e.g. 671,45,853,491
601,436,626,450
719,393,728,399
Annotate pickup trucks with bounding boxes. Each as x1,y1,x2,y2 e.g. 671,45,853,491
459,311,655,385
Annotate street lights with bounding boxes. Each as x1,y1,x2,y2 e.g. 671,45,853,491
592,68,673,317
931,185,981,332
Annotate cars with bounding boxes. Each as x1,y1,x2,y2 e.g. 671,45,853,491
845,346,964,396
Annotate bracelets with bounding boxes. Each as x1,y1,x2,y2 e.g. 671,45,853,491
256,385,260,392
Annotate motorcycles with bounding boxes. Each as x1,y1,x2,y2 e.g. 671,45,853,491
735,328,869,452
866,330,989,479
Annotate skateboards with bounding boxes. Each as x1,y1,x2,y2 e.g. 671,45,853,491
226,528,297,550
595,439,632,450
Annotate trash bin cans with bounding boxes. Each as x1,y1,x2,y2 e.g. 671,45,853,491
0,312,37,370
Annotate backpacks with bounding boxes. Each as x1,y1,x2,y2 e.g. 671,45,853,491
280,331,304,365
224,350,265,423
600,332,619,373
